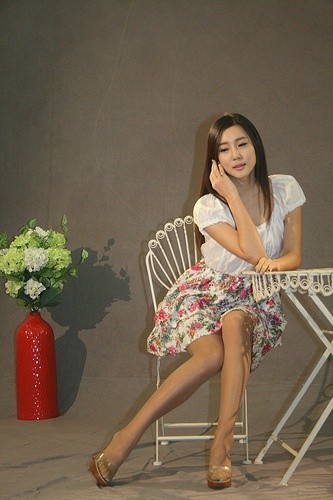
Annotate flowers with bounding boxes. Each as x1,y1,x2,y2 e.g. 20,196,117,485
0,215,89,313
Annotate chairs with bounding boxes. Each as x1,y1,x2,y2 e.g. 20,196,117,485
146,215,251,466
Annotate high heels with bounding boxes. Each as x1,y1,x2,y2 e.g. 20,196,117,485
207,465,232,489
90,430,118,487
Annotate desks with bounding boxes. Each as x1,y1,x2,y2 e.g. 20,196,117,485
241,268,333,486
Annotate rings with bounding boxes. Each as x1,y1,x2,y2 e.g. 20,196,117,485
219,172,225,176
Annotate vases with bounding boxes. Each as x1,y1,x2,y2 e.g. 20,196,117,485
15,312,58,421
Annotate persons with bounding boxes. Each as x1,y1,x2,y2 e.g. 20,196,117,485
90,113,306,489
